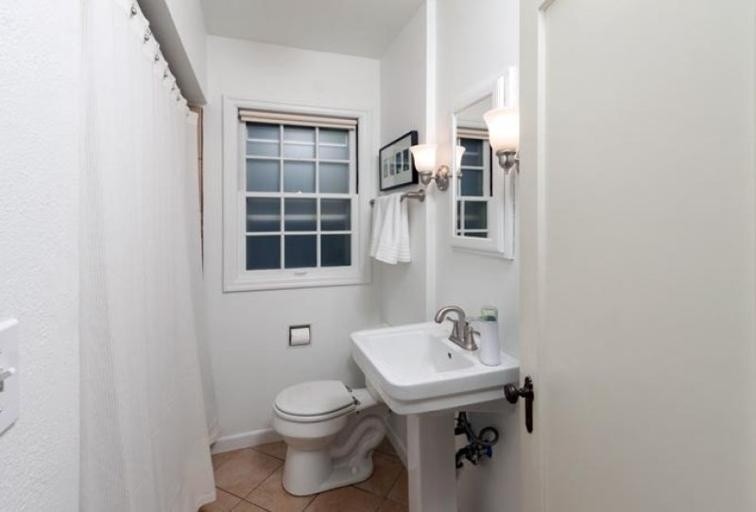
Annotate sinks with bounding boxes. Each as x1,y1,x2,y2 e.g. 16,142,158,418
349,321,521,415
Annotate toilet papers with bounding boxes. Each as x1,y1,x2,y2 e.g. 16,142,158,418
290,327,310,346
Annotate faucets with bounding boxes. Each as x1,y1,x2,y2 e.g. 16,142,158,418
435,306,469,342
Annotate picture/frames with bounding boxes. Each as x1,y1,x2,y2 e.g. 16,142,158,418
372,130,421,192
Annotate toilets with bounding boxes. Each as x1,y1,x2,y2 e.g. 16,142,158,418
273,375,388,496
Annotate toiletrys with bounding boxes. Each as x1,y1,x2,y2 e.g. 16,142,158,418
471,305,499,365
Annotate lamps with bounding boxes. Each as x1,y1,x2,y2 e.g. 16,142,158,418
409,130,442,189
482,102,516,168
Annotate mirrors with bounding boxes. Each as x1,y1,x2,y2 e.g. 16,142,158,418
409,2,541,367
442,80,509,254
